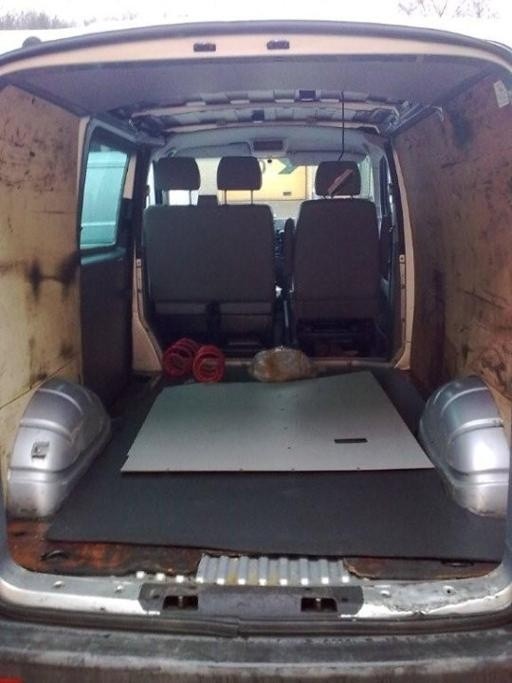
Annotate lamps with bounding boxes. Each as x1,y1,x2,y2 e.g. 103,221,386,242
211,155,276,347
290,162,382,335
151,154,211,348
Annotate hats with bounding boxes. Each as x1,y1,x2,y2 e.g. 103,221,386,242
81,148,375,264
